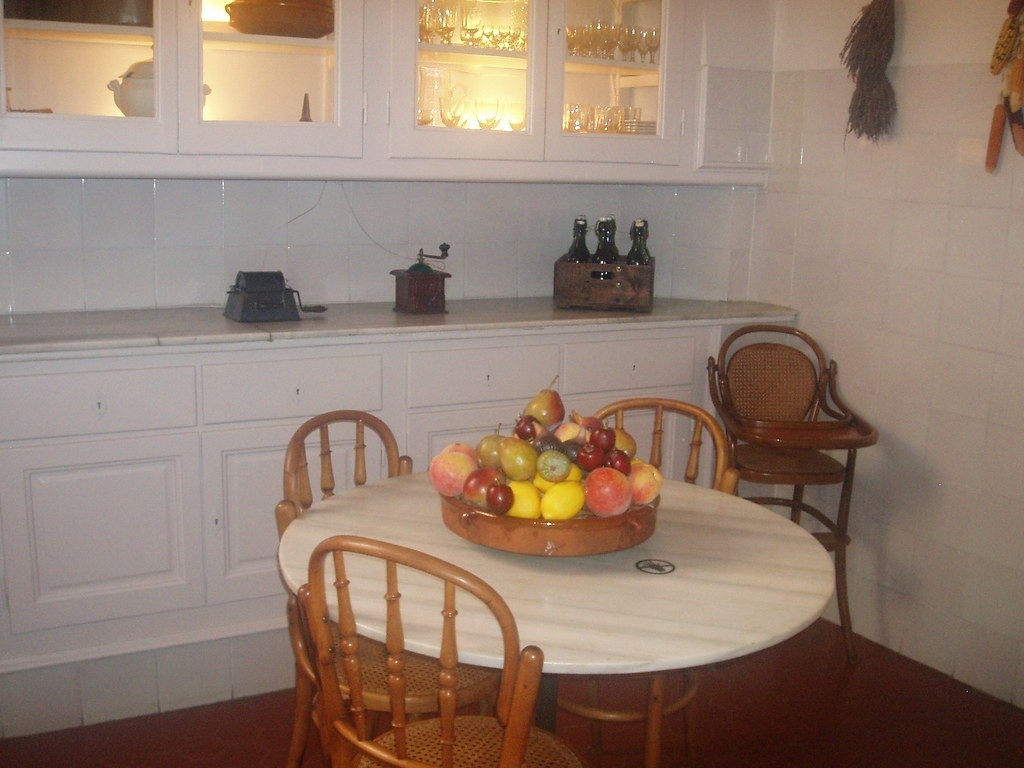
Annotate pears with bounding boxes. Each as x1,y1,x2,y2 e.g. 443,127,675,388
477,374,565,480
612,428,636,458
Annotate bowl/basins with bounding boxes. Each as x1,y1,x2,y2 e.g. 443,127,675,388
224,0,335,38
107,45,212,118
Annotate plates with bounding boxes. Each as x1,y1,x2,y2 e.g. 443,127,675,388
620,120,657,133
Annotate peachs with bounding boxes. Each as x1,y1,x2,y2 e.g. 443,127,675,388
428,443,479,497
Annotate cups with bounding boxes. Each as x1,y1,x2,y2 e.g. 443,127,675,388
417,67,526,130
564,102,641,133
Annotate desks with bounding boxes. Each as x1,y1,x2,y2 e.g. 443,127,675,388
279,470,837,768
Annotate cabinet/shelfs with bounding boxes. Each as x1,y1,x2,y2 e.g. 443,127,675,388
0,0,779,186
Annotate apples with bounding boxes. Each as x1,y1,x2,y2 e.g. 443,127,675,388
463,465,508,511
585,463,663,517
554,423,591,443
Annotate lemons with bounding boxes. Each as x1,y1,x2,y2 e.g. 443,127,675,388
507,458,584,521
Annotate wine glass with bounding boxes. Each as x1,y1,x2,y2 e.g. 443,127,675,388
565,20,660,63
418,0,529,51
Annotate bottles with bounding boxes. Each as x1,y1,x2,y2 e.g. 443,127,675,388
567,213,651,278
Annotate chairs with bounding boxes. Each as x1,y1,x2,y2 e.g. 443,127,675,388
707,323,880,666
273,408,502,768
297,533,588,768
558,399,742,768
